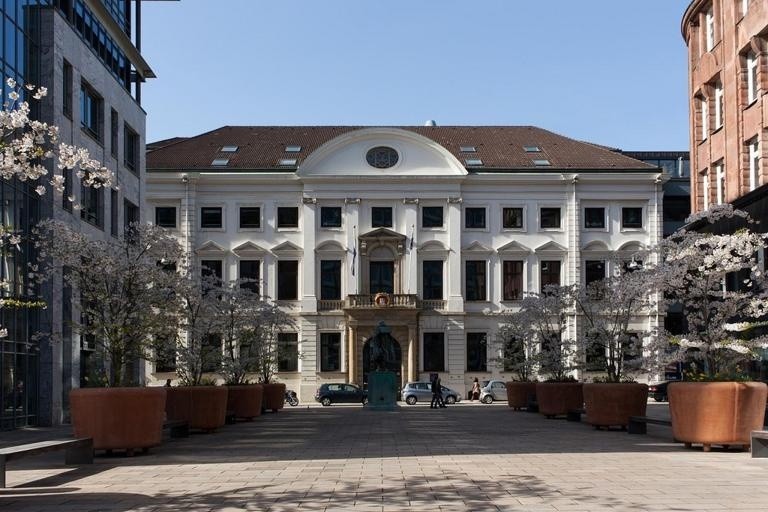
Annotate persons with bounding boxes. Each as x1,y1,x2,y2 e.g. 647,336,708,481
470,377,480,401
430,376,443,408
433,378,450,408
163,378,171,387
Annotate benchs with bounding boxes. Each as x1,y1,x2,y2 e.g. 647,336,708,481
1,436,93,488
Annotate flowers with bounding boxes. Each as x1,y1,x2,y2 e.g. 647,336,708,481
480,201,768,381
0,78,121,211
1,219,300,384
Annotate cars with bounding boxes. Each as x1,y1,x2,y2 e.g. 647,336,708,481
648,371,693,401
479,379,509,404
401,381,462,405
314,383,368,406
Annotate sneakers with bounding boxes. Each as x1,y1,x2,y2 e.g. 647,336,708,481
431,404,447,409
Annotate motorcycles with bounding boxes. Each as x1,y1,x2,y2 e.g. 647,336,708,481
284,389,299,406
